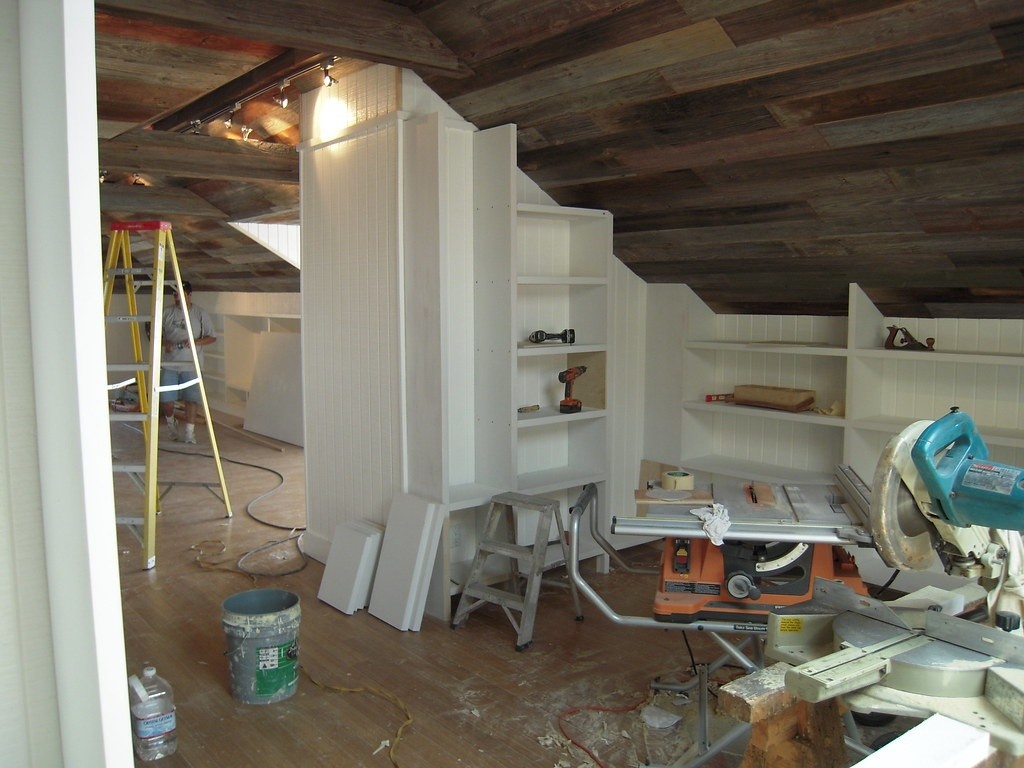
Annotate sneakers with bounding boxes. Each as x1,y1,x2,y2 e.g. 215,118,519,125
183,431,196,444
166,418,179,440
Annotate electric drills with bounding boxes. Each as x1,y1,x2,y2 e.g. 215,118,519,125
557,362,594,412
528,328,575,344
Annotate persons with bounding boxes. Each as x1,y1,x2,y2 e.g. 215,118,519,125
159,280,217,444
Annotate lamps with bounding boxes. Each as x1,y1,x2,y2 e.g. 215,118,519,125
190,55,342,134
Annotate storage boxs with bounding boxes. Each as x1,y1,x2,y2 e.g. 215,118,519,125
734,384,817,413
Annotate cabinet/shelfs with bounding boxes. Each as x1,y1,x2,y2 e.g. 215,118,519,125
405,111,615,622
685,281,1024,596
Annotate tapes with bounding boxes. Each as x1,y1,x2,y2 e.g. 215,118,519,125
661,470,695,491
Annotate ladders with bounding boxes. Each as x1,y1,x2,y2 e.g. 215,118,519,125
448,491,585,652
103,218,233,573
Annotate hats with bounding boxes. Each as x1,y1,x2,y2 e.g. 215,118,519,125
170,281,192,292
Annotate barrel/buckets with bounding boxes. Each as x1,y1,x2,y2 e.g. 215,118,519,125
222,588,302,704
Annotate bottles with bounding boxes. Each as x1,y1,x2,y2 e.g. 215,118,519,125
129,667,178,761
168,342,187,350
